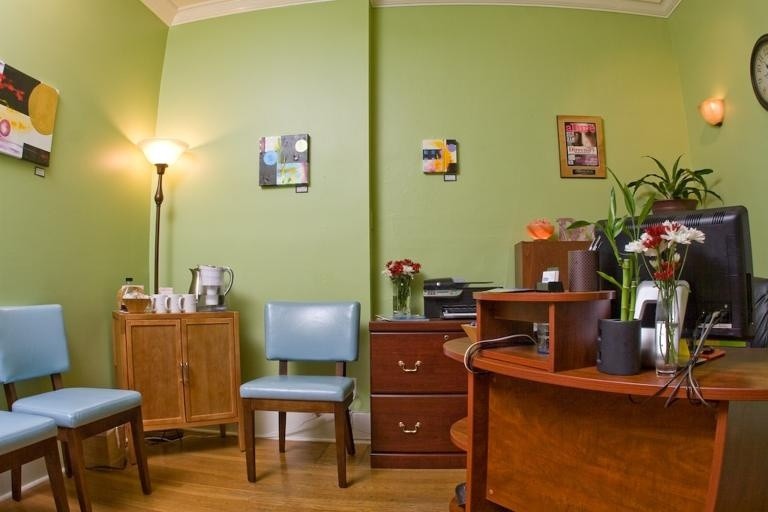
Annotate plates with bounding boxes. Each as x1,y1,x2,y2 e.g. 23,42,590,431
121,278,133,312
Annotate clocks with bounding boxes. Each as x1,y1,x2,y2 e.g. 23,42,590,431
748,32,767,115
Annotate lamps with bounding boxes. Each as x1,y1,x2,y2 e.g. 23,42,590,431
697,96,724,127
141,138,186,296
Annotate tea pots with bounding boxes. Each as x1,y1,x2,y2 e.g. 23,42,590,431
188,265,233,308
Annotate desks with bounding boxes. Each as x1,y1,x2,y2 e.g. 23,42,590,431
436,327,767,512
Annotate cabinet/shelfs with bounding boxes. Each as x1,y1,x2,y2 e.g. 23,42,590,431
110,307,246,453
370,316,475,470
514,240,590,290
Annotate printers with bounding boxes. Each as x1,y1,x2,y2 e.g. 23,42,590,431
422,278,503,320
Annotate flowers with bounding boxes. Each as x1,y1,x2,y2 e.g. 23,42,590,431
381,257,421,308
623,219,704,364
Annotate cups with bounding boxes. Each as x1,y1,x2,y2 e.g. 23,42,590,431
151,293,198,314
566,250,600,292
537,323,550,353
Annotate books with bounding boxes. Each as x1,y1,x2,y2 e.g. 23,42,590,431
376,313,430,323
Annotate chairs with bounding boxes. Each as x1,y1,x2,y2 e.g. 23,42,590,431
237,302,359,489
0,301,152,512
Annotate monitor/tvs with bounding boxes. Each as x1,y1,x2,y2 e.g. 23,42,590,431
595,205,757,367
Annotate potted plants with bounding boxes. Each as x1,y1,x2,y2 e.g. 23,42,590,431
625,153,723,214
575,169,654,376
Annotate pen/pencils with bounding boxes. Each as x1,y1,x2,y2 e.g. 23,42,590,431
588,235,605,251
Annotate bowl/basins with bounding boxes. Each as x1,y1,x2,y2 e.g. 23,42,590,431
525,224,555,241
123,298,149,313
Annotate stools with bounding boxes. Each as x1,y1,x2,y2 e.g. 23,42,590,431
0,407,71,512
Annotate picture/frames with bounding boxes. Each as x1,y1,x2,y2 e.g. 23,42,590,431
556,114,608,179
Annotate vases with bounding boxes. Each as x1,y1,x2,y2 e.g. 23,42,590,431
653,287,679,378
390,281,410,316
